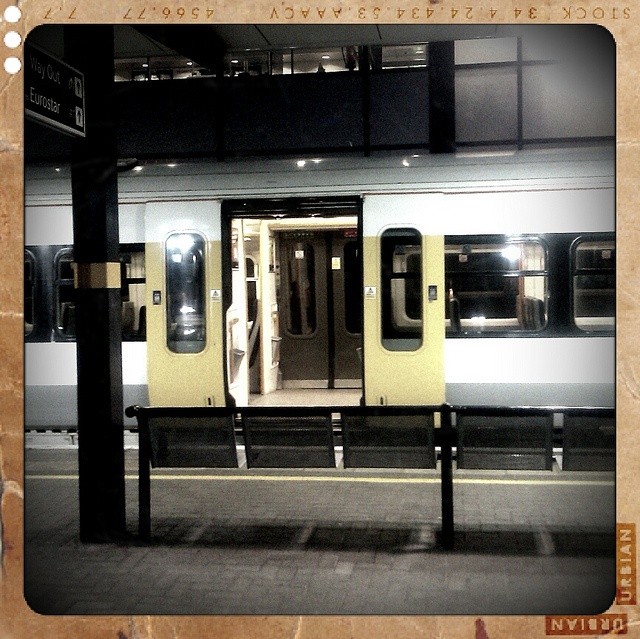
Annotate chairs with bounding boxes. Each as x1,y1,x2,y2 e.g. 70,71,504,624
456,404,553,471
147,406,247,469
562,406,615,471
242,406,343,469
340,405,438,469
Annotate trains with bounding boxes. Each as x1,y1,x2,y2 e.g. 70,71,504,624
5,141,618,432
